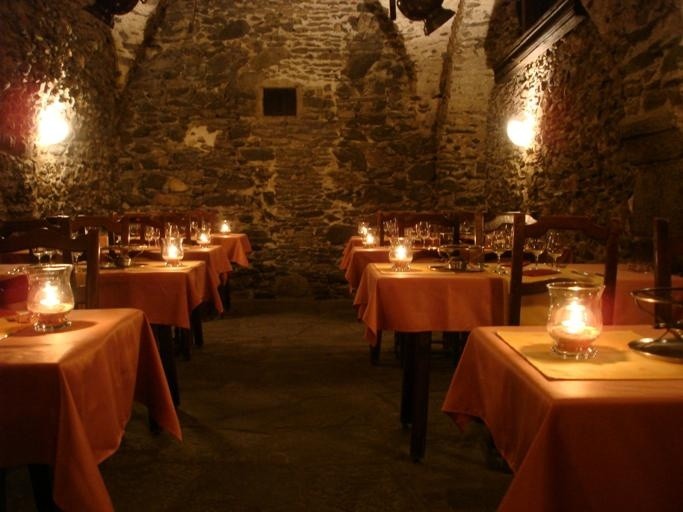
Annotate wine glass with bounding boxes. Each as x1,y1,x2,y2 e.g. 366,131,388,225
348,215,567,276
545,282,605,360
22,215,232,335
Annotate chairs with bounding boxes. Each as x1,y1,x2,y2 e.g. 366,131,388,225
337,211,683,512
0,205,252,511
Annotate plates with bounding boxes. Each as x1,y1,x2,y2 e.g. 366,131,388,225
627,337,681,365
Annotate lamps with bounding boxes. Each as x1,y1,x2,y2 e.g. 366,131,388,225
389,1,455,37
